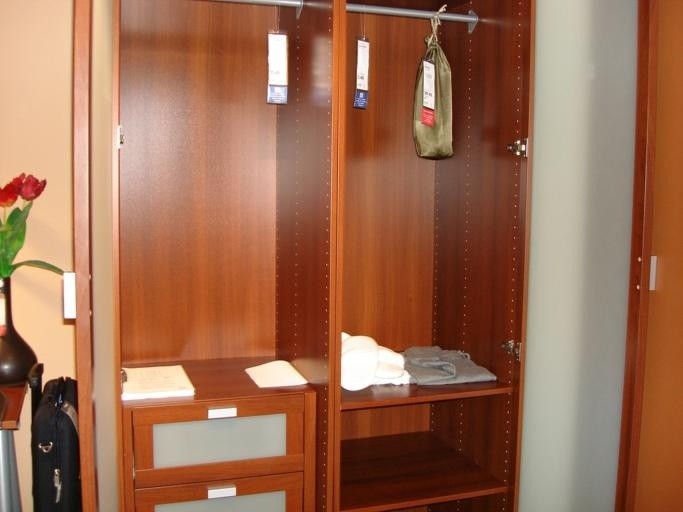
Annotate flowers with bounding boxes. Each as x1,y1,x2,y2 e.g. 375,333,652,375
0,173,65,278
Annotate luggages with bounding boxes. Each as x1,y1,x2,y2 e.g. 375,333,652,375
31,377,81,512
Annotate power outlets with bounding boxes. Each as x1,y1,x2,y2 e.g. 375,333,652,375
61,271,76,322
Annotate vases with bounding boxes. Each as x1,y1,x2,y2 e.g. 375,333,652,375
1,278,37,429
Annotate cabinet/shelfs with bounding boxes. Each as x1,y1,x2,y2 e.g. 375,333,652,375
116,0,337,512
337,0,534,512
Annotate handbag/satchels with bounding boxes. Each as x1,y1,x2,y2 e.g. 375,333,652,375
413,6,454,161
